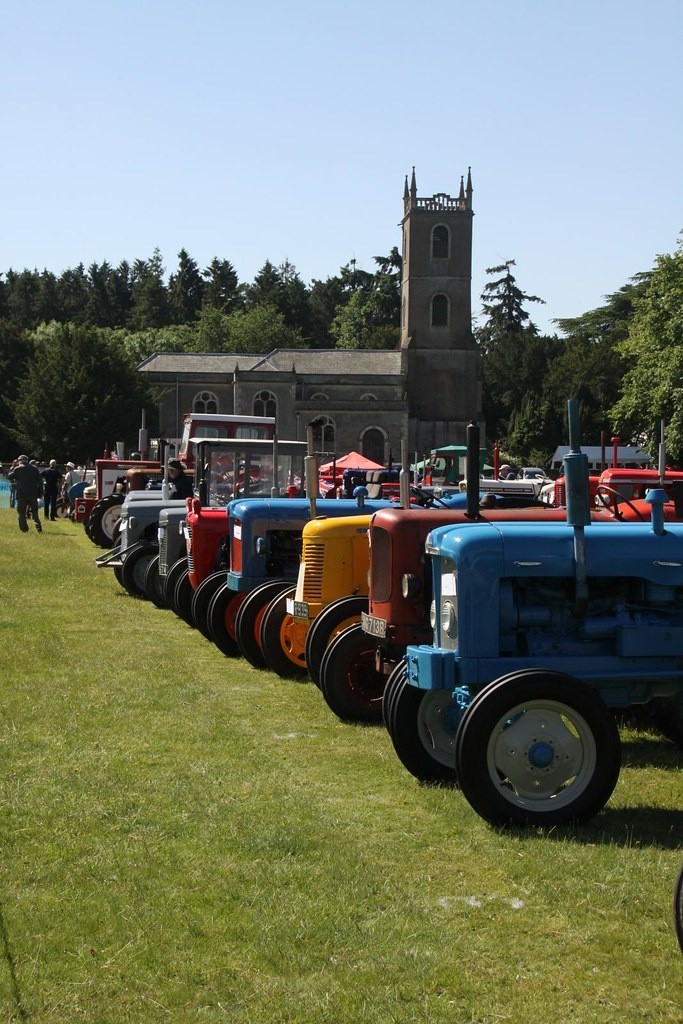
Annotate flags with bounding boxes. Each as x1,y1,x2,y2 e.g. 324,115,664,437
293,474,335,498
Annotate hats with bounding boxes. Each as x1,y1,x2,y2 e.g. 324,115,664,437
28,460,36,465
18,455,29,461
64,462,75,469
499,464,510,471
479,494,501,506
160,458,188,471
49,459,56,466
506,473,515,480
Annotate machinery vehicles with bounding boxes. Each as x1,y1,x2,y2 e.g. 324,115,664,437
280,433,683,723
206,434,554,674
70,405,682,611
383,400,683,831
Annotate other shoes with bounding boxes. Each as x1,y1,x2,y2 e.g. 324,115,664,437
37,528,42,533
44,516,49,520
20,526,29,533
50,518,56,521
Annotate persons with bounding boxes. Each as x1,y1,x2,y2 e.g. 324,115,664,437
8,455,81,534
161,457,194,499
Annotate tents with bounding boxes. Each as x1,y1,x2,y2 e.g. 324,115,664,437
319,450,386,475
